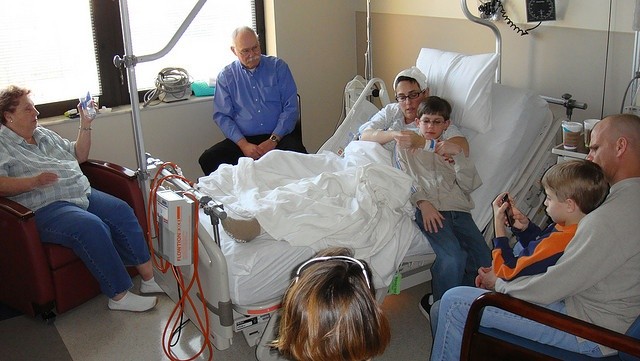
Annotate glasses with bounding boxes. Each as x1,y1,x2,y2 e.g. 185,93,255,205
296,256,370,289
419,118,446,126
239,45,260,56
396,89,426,102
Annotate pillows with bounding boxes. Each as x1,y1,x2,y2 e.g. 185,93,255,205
413,46,499,135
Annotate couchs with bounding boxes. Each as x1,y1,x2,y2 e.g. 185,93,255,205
456,279,640,361
0,157,151,324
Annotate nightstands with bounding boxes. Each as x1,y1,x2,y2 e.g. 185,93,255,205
552,135,594,167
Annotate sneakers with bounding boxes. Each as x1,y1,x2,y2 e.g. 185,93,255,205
418,293,433,321
140,280,164,294
108,291,158,312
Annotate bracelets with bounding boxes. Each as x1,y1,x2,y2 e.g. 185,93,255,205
78,124,92,130
425,139,437,153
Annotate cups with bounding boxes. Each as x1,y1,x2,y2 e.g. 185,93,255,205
561,120,583,149
583,118,600,146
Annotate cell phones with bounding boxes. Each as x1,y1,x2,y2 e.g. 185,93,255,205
498,194,516,228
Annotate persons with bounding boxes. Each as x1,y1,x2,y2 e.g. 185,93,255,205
491,160,610,282
358,67,470,169
267,246,391,361
397,95,493,321
0,86,167,311
199,26,309,176
428,114,638,358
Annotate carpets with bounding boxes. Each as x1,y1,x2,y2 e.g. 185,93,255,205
52,272,259,361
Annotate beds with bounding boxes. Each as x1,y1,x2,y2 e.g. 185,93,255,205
144,76,588,361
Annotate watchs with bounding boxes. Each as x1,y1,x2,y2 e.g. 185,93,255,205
269,135,280,144
